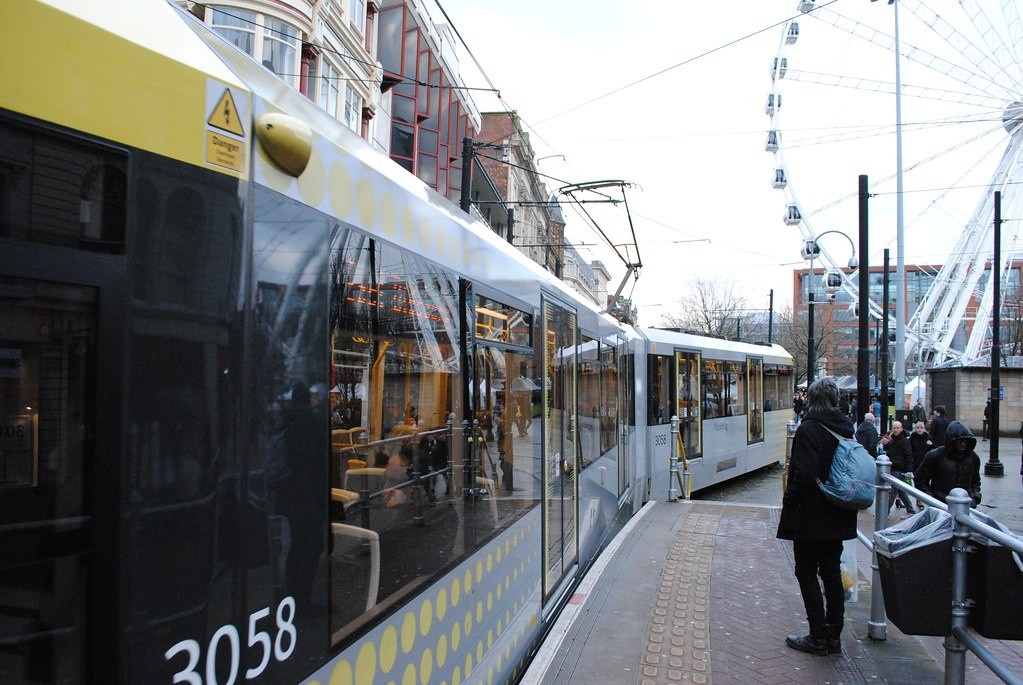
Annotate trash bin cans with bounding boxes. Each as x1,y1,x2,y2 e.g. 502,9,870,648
967,507,1023,641
872,505,952,637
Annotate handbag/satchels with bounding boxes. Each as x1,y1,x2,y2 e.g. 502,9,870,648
816,538,859,603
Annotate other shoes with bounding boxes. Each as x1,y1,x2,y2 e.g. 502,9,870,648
901,512,914,520
917,501,925,510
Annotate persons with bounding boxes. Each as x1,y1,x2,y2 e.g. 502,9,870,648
793,389,808,423
912,398,928,425
856,413,879,459
871,398,881,434
910,422,937,510
776,378,855,656
984,401,991,439
914,421,982,508
330,391,532,523
883,421,916,514
838,393,894,423
929,405,950,447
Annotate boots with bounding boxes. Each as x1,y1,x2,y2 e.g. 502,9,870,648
785,620,828,656
825,622,846,653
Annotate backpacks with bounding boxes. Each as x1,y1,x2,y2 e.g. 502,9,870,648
805,418,878,511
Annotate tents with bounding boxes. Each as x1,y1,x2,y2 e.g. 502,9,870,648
797,374,895,397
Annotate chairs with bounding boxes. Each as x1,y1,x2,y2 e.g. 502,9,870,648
330,423,411,638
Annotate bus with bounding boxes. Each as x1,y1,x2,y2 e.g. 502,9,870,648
1,0,792,685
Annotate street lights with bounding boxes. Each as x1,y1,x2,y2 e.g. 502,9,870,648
807,230,857,390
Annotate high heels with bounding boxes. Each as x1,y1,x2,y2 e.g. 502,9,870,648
895,498,905,509
